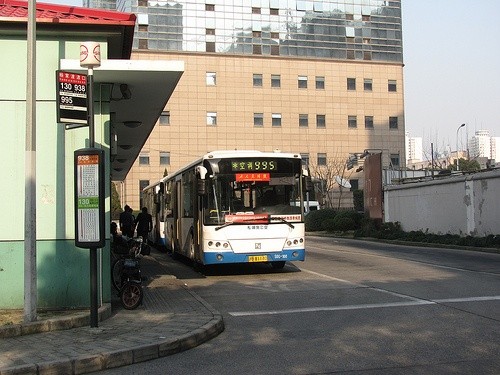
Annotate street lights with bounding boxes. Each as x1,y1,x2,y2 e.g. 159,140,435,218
456,123,465,171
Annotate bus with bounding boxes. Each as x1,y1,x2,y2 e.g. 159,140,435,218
143,181,162,249
162,149,314,273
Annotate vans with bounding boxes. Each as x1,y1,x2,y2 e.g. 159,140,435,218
288,201,320,218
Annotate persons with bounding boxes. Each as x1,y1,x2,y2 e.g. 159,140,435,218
120,204,134,237
111,221,118,235
133,207,153,244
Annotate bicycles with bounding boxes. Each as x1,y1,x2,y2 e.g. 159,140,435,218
109,237,147,311
